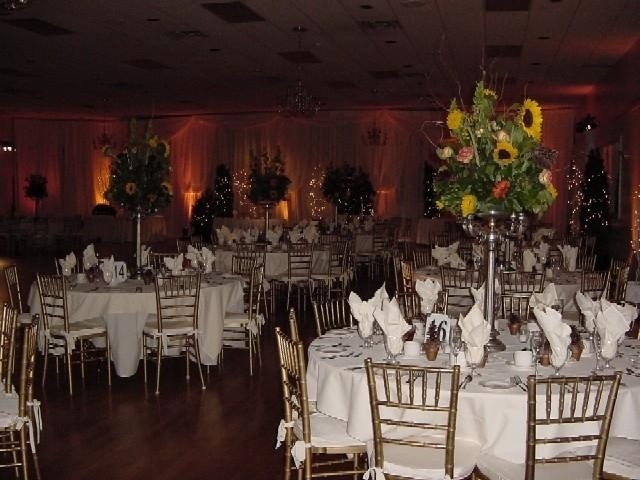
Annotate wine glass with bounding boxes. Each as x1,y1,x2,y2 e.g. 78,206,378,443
60,252,213,288
217,233,318,252
356,300,630,378
430,242,578,277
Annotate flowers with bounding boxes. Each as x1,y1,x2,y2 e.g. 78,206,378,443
420,81,560,216
100,118,174,212
248,145,291,204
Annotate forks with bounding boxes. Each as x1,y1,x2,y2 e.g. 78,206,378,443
509,375,529,391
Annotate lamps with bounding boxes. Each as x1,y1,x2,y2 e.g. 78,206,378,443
277,25,323,116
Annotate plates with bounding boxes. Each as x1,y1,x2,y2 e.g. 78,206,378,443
317,327,640,389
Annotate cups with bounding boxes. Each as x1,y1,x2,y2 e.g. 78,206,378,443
77,273,86,280
